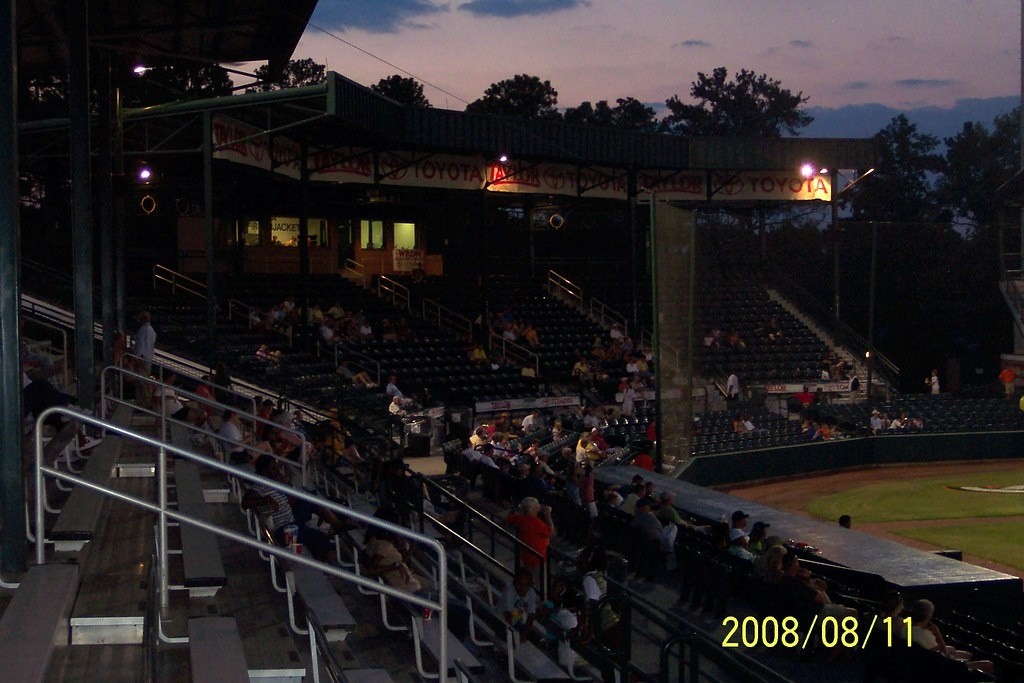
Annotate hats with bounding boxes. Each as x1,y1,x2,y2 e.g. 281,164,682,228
752,521,770,529
731,511,749,521
538,450,550,456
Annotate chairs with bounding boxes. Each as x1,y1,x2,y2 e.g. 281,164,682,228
128,214,1024,683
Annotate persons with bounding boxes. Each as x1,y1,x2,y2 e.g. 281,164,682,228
20,297,1024,683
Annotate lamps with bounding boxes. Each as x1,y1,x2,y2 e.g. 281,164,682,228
488,151,512,163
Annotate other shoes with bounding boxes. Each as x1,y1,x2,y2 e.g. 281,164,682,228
61,409,88,424
79,435,101,449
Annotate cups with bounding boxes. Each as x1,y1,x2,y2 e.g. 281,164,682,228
291,544,303,556
424,607,432,621
285,528,297,548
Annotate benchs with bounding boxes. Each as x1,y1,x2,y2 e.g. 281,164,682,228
0,341,624,683
